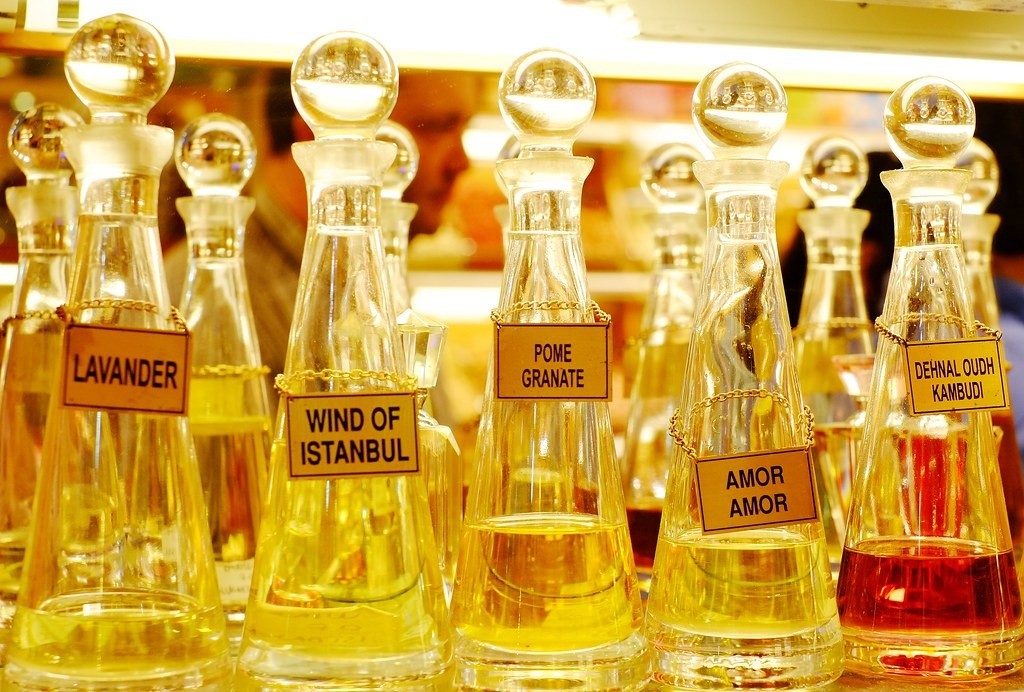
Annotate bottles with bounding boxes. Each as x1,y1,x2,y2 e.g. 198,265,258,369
645,60,845,692
398,309,464,609
836,77,1024,684
952,137,1023,548
5,13,232,692
235,30,456,692
620,143,708,591
376,118,419,344
0,103,79,627
793,137,873,593
174,114,274,656
448,49,650,692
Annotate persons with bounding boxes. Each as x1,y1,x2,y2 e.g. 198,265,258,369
766,149,912,333
969,99,1024,457
146,62,480,377
